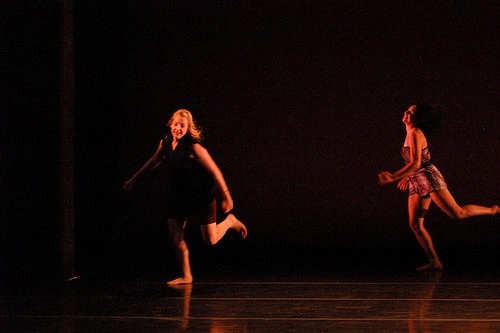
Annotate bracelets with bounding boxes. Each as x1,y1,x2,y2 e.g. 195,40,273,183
221,190,229,194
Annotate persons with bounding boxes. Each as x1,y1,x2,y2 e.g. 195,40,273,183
123,109,248,286
379,101,500,272
